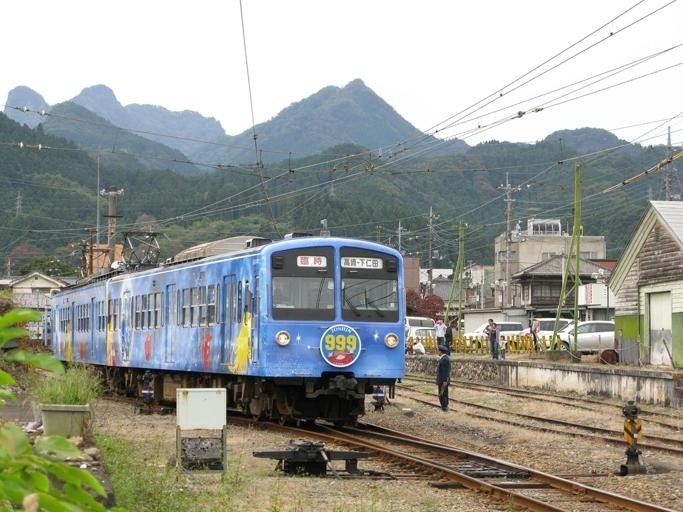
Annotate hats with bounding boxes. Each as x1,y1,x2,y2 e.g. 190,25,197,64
438,345,449,352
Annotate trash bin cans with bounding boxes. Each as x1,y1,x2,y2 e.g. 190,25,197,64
38,402,93,441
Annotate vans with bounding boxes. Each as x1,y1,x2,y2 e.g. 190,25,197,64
468,321,522,349
405,316,436,327
528,317,581,349
456,319,464,334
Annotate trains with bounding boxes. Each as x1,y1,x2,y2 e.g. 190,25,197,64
47,230,406,429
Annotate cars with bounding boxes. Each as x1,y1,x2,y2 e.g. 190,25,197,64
518,328,530,348
550,320,614,352
404,326,436,354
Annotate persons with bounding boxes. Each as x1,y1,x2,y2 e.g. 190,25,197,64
433,319,508,360
411,335,426,356
435,344,452,412
